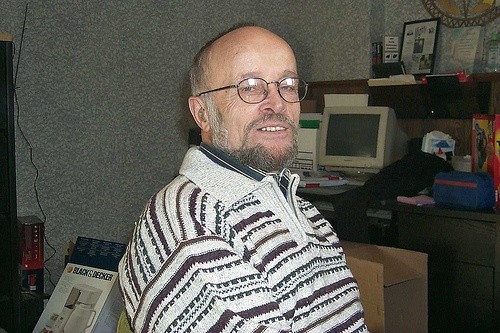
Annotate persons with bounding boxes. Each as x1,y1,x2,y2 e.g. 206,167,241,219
119,23,370,333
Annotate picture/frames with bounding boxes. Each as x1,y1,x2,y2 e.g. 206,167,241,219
400,17,441,75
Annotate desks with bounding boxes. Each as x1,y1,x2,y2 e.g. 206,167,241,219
298,177,500,333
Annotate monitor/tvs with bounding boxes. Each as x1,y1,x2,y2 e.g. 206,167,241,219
318,107,412,185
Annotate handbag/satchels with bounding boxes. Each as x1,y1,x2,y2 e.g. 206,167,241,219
432,173,495,209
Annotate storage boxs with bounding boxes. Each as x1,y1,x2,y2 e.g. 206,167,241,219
471,113,499,211
341,241,429,333
33,237,125,333
18,215,44,294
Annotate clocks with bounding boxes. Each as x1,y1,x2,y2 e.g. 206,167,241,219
422,0,500,29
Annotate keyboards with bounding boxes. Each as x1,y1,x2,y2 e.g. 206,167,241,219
310,198,336,212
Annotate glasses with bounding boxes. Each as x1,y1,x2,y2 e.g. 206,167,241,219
196,77,310,103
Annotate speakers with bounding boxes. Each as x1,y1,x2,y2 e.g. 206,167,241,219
0,33,22,333
